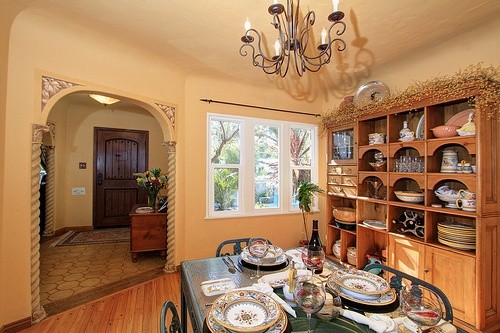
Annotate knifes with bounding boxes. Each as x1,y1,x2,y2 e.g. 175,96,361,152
228,256,243,273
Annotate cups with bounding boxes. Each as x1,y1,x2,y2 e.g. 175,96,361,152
368,133,385,145
459,189,476,200
400,130,415,139
316,285,342,320
456,199,476,211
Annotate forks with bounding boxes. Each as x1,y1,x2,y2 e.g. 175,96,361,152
222,258,236,274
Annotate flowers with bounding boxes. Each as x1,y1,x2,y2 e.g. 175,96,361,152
137,168,167,190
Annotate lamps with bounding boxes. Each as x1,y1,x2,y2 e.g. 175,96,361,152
239,0,347,78
89,93,120,106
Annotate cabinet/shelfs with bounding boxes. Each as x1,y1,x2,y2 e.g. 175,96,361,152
130,202,167,260
326,80,500,333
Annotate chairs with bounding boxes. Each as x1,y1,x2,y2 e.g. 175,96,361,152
363,263,452,323
216,237,270,255
160,301,184,333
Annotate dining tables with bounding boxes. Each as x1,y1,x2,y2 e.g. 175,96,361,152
181,249,457,333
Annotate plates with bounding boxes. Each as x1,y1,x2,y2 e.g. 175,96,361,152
206,268,396,333
257,270,312,287
238,245,289,273
445,109,477,128
363,219,387,230
437,221,476,250
434,186,464,208
398,138,414,142
416,114,424,139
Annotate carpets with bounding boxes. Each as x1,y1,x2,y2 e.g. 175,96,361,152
55,226,130,245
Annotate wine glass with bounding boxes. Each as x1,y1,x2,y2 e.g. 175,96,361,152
293,275,326,333
301,245,325,276
400,285,442,333
248,237,269,282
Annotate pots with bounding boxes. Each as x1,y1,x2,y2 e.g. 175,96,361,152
428,125,460,137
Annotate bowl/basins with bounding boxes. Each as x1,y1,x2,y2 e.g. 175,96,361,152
394,191,424,202
331,208,356,231
470,166,475,173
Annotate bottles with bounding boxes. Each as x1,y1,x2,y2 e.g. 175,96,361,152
399,121,412,137
331,240,340,258
306,219,323,274
440,149,472,174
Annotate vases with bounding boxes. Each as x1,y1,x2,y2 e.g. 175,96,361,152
146,193,156,209
